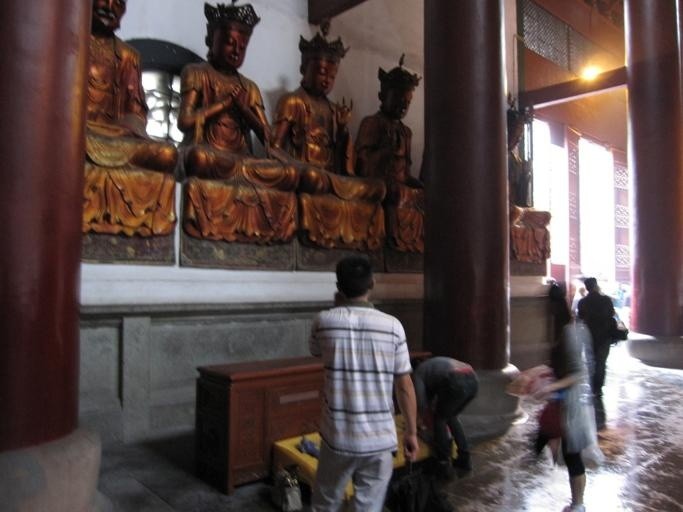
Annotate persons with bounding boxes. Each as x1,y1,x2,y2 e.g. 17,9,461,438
352,53,426,254
82,0,179,236
308,256,419,512
507,91,551,262
272,31,386,250
519,285,604,512
578,288,585,297
409,356,480,481
576,278,616,430
177,0,300,244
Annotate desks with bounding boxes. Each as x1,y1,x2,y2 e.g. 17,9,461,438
194,349,432,495
